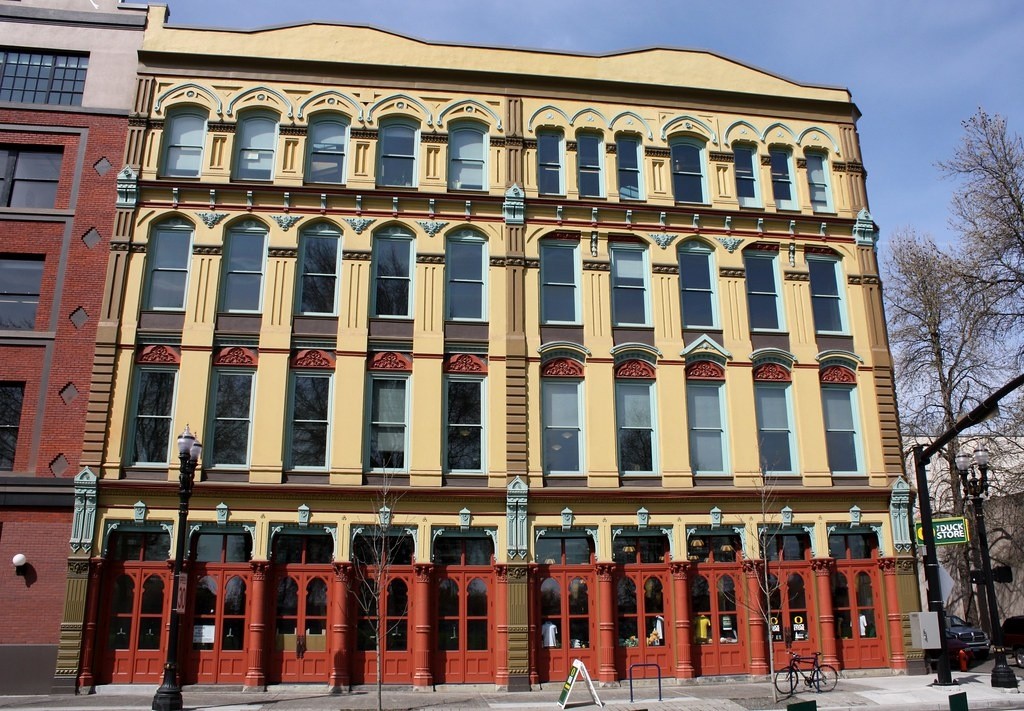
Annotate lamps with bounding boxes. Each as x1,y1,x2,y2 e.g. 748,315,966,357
459,507,471,532
12,554,27,576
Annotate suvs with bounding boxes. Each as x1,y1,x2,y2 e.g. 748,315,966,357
999,614,1024,669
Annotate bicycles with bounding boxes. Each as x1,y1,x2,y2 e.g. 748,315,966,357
774,651,839,694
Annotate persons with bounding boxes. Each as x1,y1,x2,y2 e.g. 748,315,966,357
542,621,558,648
692,615,711,641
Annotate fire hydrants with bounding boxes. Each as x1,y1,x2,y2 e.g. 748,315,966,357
958,648,969,673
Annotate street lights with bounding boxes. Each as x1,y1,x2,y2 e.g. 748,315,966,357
149,424,205,711
954,441,1018,689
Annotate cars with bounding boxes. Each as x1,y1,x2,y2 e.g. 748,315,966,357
946,630,976,667
945,615,992,663
